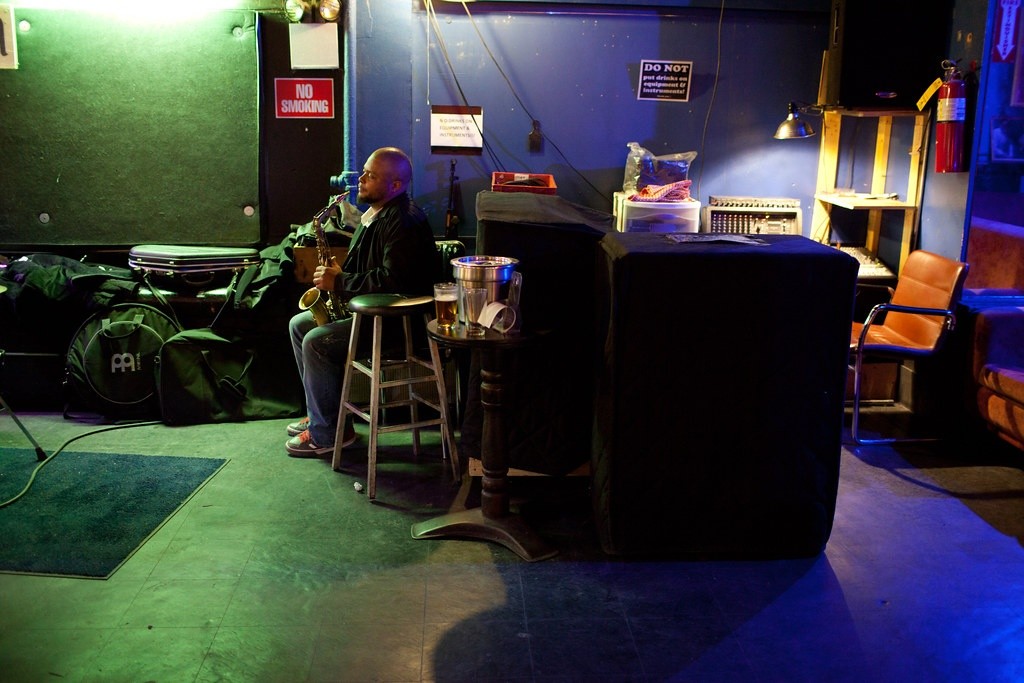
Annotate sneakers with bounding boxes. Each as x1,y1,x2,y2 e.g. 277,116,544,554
286,426,356,456
287,417,310,435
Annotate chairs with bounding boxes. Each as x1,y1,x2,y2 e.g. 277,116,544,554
846,249,970,446
366,239,465,426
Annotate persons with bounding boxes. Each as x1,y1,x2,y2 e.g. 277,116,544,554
285,146,442,457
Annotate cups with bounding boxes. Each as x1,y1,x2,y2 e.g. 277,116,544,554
463,288,487,336
433,283,459,334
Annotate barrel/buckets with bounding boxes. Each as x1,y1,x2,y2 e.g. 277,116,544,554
450,255,521,323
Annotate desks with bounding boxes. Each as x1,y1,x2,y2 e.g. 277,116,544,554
411,318,562,562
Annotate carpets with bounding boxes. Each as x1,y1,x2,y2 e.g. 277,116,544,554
0,446,232,581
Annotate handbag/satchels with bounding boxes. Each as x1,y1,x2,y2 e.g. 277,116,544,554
67,304,183,419
154,328,303,426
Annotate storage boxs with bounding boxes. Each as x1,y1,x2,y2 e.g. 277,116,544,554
492,171,557,196
612,192,701,234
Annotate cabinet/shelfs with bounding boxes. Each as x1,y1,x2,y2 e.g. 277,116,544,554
815,104,932,420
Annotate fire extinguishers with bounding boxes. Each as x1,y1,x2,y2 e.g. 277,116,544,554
935,60,966,174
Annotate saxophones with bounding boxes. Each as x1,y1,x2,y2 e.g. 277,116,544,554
297,190,352,326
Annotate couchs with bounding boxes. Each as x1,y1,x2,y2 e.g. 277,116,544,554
969,308,1024,450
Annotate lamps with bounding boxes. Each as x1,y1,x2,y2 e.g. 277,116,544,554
773,103,828,139
284,0,343,22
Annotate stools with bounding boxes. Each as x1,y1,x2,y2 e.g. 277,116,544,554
333,295,461,501
